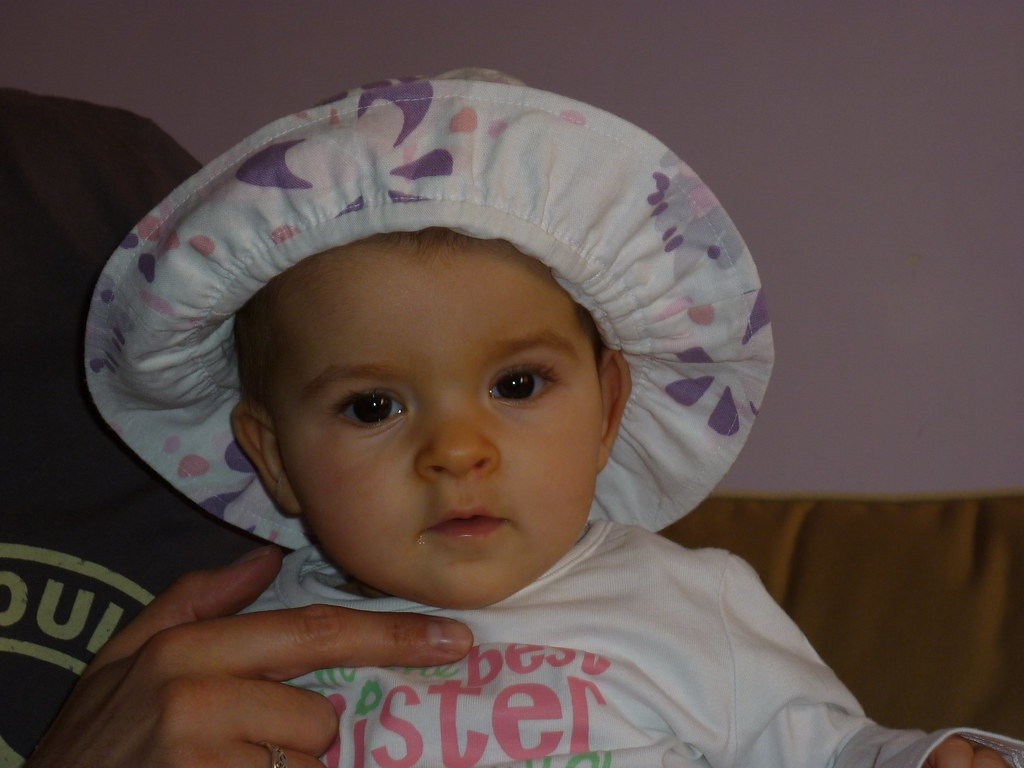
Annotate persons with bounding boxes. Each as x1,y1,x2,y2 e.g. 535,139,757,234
85,65,1024,768
1,82,477,768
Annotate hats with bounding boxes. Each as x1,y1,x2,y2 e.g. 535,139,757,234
85,67,775,551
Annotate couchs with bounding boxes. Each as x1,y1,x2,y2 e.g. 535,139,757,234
653,489,1024,742
